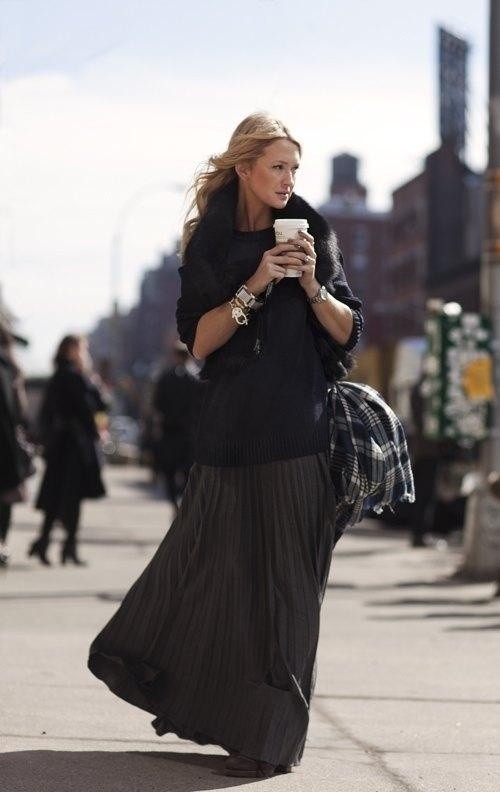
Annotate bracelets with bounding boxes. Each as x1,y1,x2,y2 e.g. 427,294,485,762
229,284,264,326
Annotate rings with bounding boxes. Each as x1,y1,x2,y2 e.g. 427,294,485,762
311,241,315,246
303,255,311,263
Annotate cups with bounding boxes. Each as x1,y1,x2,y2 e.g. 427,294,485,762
273,218,310,278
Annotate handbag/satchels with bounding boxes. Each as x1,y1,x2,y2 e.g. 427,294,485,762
322,375,417,545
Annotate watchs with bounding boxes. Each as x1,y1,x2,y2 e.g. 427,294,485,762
307,284,328,305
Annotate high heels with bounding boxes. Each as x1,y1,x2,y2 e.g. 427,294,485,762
26,533,88,568
221,751,293,780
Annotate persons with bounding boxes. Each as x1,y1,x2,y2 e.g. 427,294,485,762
172,110,368,779
